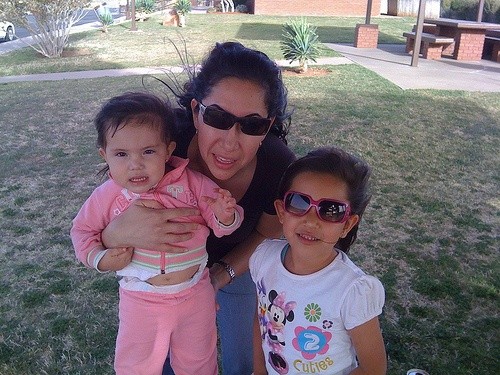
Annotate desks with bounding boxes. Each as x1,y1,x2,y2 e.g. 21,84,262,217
423,17,500,62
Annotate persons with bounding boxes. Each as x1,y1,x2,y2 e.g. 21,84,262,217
98,39,299,375
69,93,245,375
248,147,388,375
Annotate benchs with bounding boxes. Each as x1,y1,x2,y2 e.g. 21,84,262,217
403,31,455,60
411,24,437,54
484,36,500,64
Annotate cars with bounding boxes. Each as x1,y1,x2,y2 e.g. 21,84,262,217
0,9,15,42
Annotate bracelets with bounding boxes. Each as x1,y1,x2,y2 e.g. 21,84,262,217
216,259,236,280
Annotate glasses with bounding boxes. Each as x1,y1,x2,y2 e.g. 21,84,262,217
282,190,351,223
198,101,272,136
